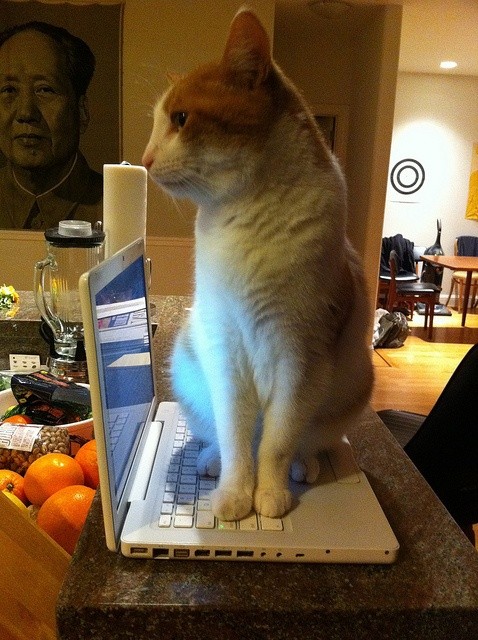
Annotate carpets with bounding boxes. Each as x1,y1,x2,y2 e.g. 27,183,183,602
376,408,428,450
417,302,452,317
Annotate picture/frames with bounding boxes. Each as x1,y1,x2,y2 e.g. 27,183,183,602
0,1,123,235
309,103,348,176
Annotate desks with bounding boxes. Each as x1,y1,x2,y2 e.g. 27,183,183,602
1,292,478,637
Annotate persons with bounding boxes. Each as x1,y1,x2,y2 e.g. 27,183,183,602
1,21,103,231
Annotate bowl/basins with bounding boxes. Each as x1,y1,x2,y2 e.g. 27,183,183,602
0,383,93,441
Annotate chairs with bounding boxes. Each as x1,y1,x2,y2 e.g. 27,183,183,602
445,236,478,315
403,341,477,550
384,250,441,340
375,271,420,320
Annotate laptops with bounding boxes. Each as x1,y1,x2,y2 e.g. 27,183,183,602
77,237,402,568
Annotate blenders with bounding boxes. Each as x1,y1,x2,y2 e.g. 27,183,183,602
33,220,106,382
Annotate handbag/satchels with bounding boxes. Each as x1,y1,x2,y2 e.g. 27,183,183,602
373,313,399,348
386,312,409,348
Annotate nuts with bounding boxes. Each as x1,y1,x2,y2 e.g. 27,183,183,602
0,422,70,477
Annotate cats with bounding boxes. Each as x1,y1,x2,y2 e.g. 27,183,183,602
141,5,374,521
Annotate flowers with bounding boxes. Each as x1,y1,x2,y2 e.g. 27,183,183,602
0,283,20,310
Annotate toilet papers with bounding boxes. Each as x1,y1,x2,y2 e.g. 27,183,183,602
102,163,148,260
